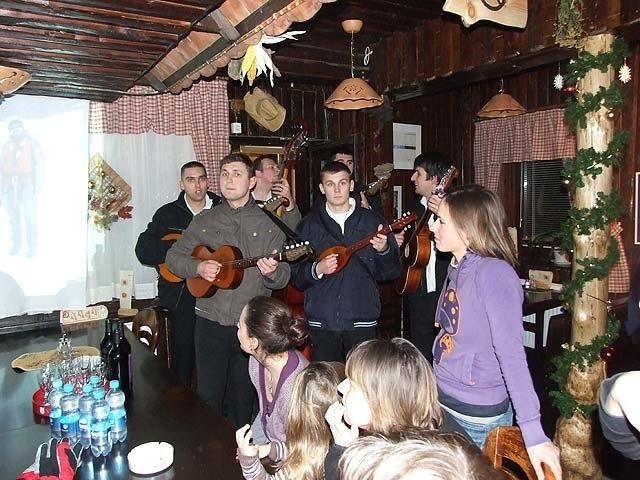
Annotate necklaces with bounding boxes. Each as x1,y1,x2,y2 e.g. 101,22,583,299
264,350,285,394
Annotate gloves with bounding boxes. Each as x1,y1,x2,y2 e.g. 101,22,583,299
17,438,84,480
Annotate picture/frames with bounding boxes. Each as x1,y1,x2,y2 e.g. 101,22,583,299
394,186,402,222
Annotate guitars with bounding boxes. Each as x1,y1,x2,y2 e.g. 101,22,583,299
274,121,307,220
186,240,314,298
156,197,290,283
393,164,458,296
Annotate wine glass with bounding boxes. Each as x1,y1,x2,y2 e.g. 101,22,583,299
37,372,53,408
42,358,108,392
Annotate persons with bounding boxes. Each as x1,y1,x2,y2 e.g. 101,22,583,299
289,160,405,369
427,182,563,480
133,146,639,480
163,153,292,432
0,119,46,261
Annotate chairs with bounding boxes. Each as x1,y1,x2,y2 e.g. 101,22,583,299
484,427,555,480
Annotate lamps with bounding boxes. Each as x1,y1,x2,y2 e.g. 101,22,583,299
477,78,528,119
323,20,385,112
0,64,29,101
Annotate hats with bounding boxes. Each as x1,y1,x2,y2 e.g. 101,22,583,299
243,87,287,132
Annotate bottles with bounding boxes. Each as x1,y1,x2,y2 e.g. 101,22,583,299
79,385,95,449
88,378,106,401
48,383,63,440
59,387,78,445
91,389,110,458
104,380,128,445
101,319,133,392
